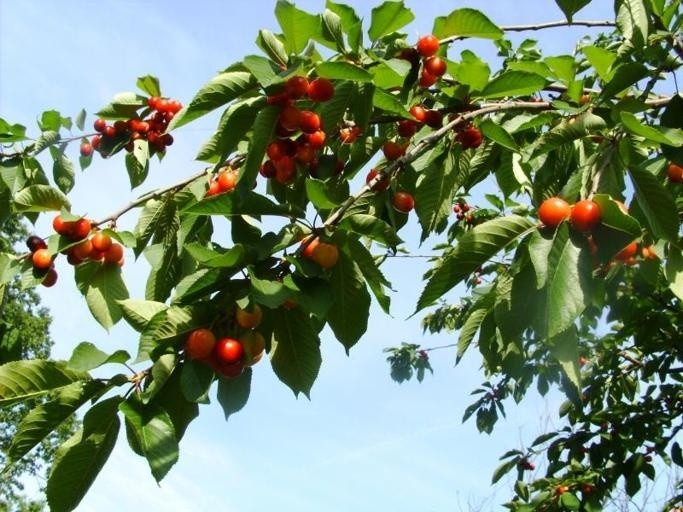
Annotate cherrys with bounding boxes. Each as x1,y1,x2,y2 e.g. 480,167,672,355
25,35,683,495
